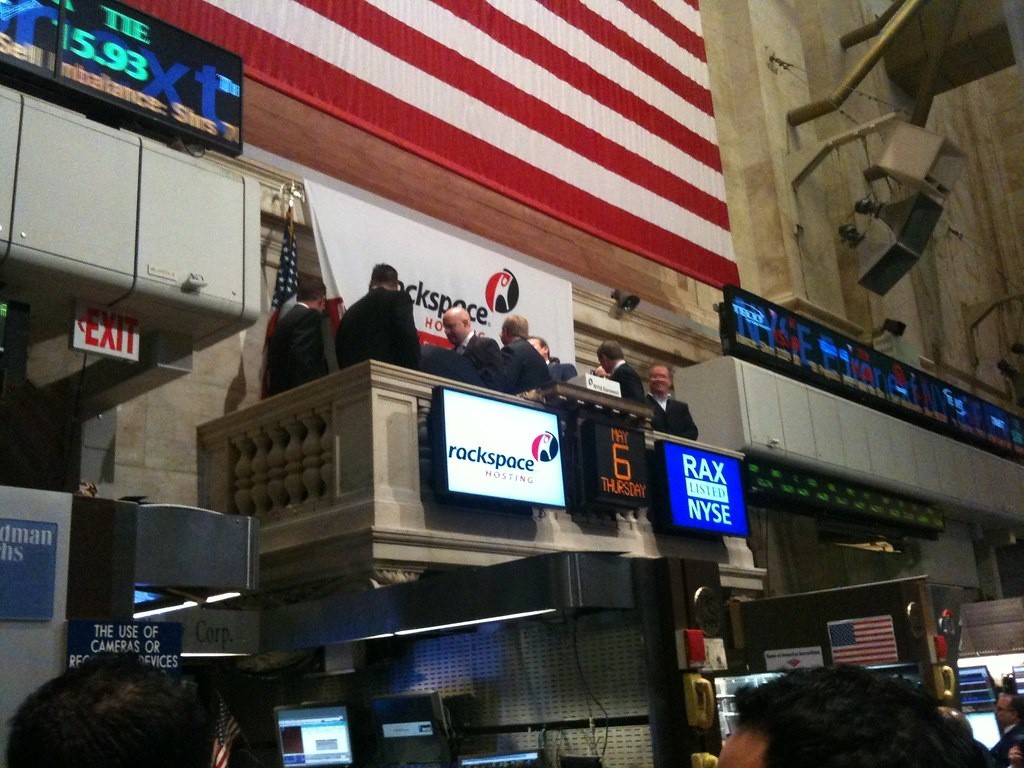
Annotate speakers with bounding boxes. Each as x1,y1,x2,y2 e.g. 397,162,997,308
875,122,968,200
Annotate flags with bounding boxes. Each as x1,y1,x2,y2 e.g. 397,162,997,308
263,207,300,349
210,697,242,768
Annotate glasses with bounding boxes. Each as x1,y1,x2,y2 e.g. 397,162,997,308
499,332,503,337
997,706,1010,712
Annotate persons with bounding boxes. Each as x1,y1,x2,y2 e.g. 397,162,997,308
716,661,1024,768
6,655,216,768
442,306,578,397
334,264,421,374
261,274,331,399
594,340,699,441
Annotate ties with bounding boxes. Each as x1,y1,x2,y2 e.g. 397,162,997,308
456,345,461,354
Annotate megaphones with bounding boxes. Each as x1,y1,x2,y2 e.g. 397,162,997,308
855,191,943,255
839,222,921,296
998,359,1024,408
612,289,641,311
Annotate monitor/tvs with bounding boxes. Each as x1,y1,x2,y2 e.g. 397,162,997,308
273,701,354,768
458,748,546,768
654,439,752,539
427,385,566,510
